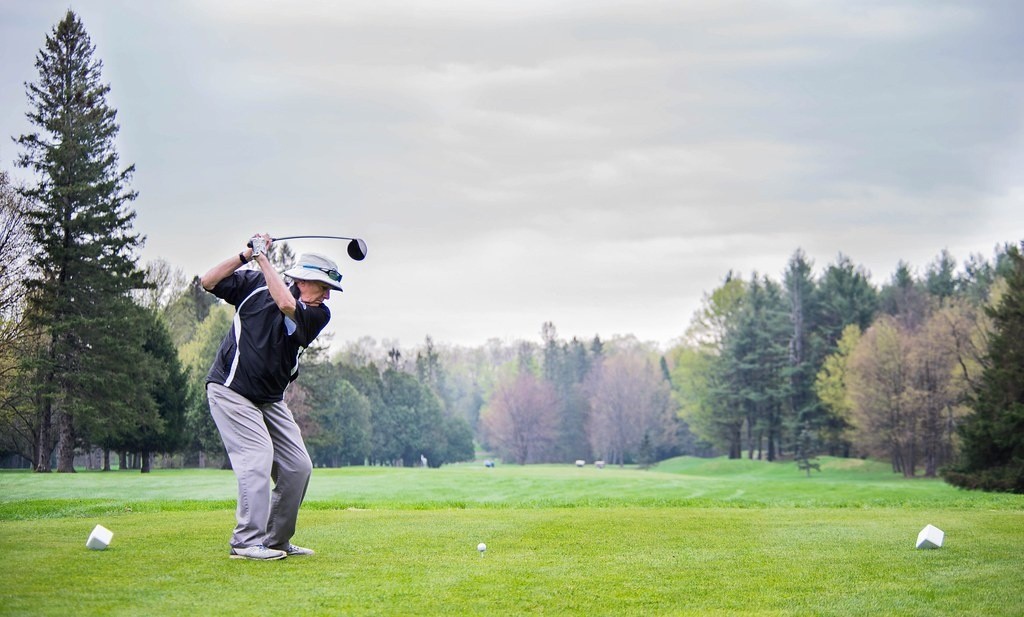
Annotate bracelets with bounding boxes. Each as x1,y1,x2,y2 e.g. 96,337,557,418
239,251,248,264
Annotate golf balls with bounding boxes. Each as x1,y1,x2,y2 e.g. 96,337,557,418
477,542,487,552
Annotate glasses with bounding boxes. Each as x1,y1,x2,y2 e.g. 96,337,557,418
303,262,343,283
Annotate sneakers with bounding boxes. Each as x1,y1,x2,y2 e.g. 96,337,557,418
283,544,315,556
230,546,287,561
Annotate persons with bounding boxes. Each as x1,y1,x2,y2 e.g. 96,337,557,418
202,234,343,561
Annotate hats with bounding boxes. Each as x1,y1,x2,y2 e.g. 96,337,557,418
284,253,343,292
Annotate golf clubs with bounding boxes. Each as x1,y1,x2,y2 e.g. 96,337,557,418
247,235,368,262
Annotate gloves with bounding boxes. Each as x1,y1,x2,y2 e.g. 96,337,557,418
249,237,268,260
